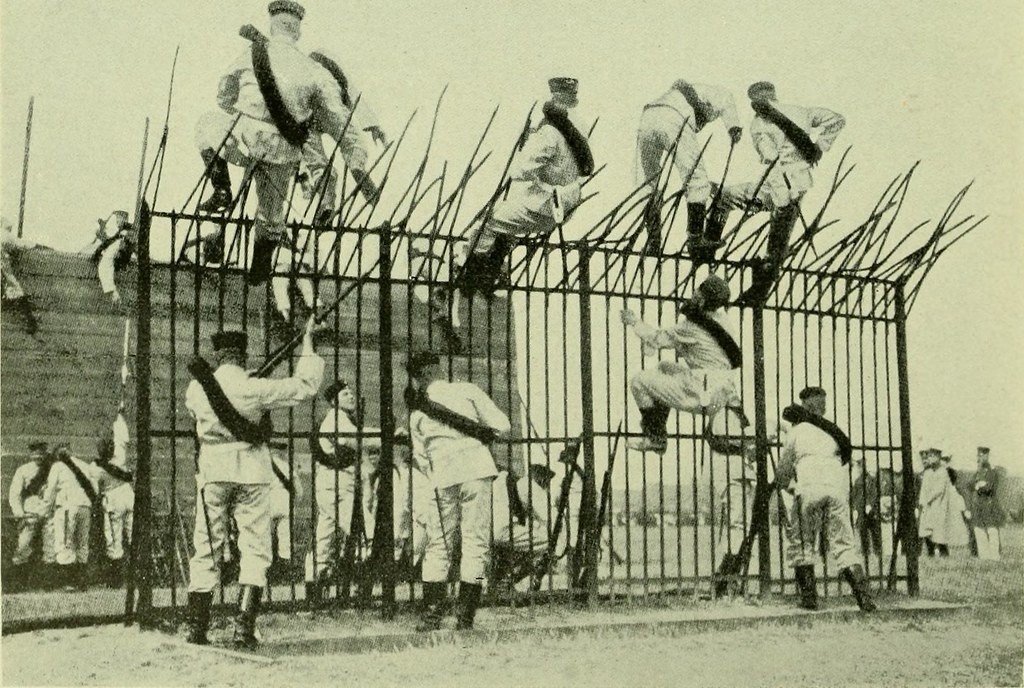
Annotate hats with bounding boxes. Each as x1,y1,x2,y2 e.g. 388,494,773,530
267,1,304,16
700,276,731,306
29,438,115,458
919,448,951,462
531,463,555,480
269,440,288,450
978,447,990,456
211,331,248,351
549,77,578,94
267,377,416,456
800,386,827,400
405,351,440,378
748,80,775,94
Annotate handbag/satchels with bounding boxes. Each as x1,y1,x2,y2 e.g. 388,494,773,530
680,299,743,369
671,78,708,132
307,52,350,108
507,474,527,525
418,392,494,445
252,38,311,147
542,101,595,177
750,98,823,167
187,355,274,446
22,454,134,503
93,236,134,270
271,425,404,490
781,402,853,465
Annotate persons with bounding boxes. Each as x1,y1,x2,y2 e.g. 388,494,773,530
456,77,595,300
408,247,463,353
198,0,393,340
300,350,562,632
0,219,56,334
185,313,329,650
619,278,748,454
88,401,134,589
699,83,845,303
9,436,98,593
633,75,744,259
95,211,138,307
850,446,1008,565
768,386,882,619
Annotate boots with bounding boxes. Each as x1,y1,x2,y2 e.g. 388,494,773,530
716,552,739,600
186,584,263,651
5,552,127,592
416,581,483,631
18,296,38,334
268,555,410,608
843,564,878,611
794,563,822,612
493,557,531,605
199,146,799,353
626,402,671,453
